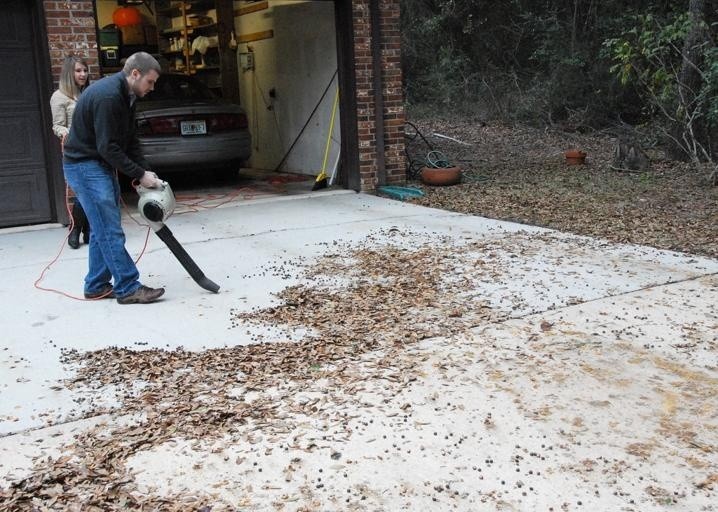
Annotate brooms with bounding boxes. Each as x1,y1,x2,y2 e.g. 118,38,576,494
311,88,340,192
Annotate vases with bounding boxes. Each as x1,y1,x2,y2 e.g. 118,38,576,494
420,163,460,186
566,151,587,167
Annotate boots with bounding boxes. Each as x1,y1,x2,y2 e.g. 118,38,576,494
69,201,84,248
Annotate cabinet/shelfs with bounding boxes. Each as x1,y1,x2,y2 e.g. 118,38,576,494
153,0,241,105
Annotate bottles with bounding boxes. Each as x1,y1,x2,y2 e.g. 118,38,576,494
168,30,196,51
175,56,183,69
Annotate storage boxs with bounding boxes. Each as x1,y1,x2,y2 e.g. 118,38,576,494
97,21,159,67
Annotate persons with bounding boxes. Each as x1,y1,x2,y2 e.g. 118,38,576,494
60,50,169,305
48,55,93,250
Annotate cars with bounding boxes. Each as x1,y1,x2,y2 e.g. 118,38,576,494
133,72,254,178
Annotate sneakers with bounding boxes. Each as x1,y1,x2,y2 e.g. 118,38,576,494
85,281,116,297
118,286,164,303
